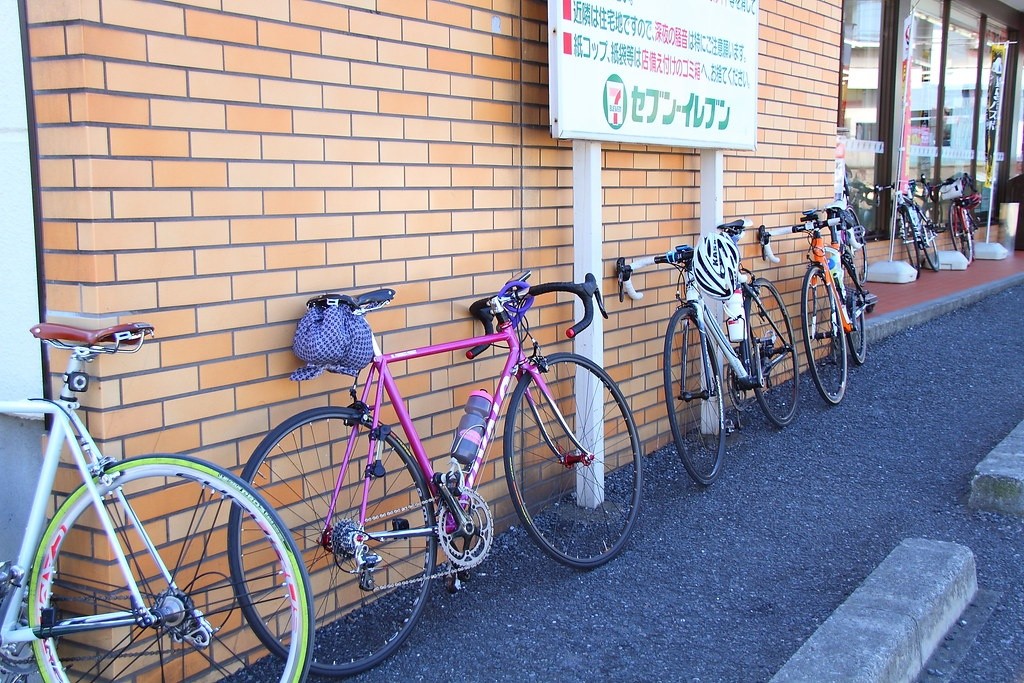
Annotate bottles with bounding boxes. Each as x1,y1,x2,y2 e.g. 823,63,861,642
724,289,748,343
825,257,846,300
911,210,920,227
451,389,493,465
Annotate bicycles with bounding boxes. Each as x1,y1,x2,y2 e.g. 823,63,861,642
846,179,878,234
0,321,315,683
874,180,941,280
836,200,867,287
757,204,867,406
226,270,643,676
616,218,799,487
917,174,981,265
